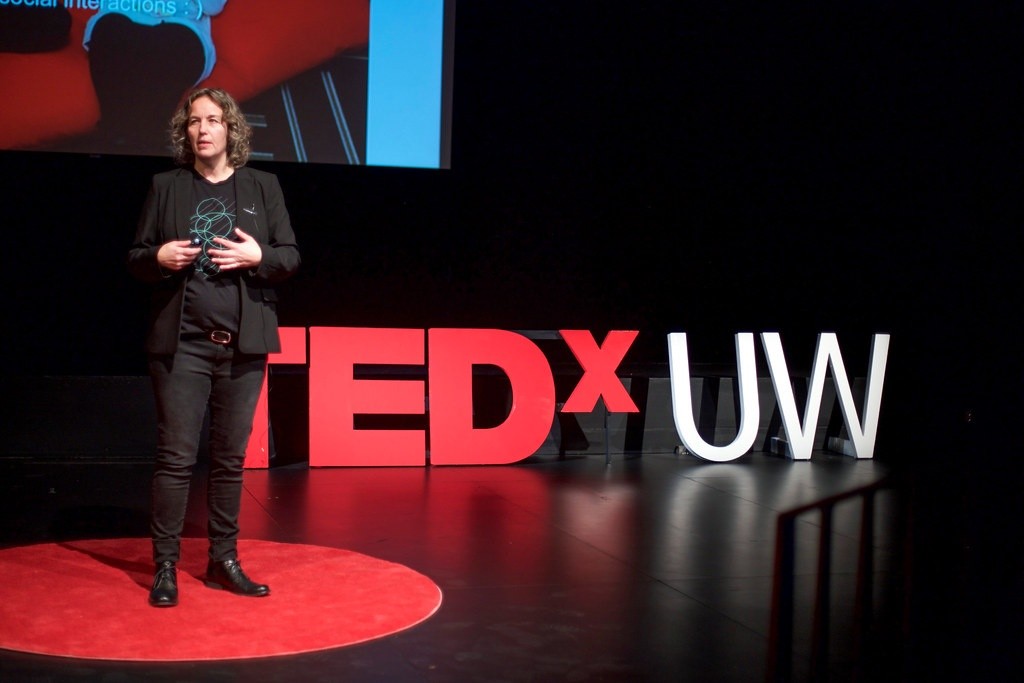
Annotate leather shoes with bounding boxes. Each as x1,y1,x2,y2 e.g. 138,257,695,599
151,561,178,606
207,556,269,596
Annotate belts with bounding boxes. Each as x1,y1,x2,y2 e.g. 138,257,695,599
196,329,238,346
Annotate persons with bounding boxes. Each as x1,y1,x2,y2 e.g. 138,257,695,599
127,88,301,606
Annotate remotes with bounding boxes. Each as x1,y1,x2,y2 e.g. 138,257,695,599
188,238,202,249
